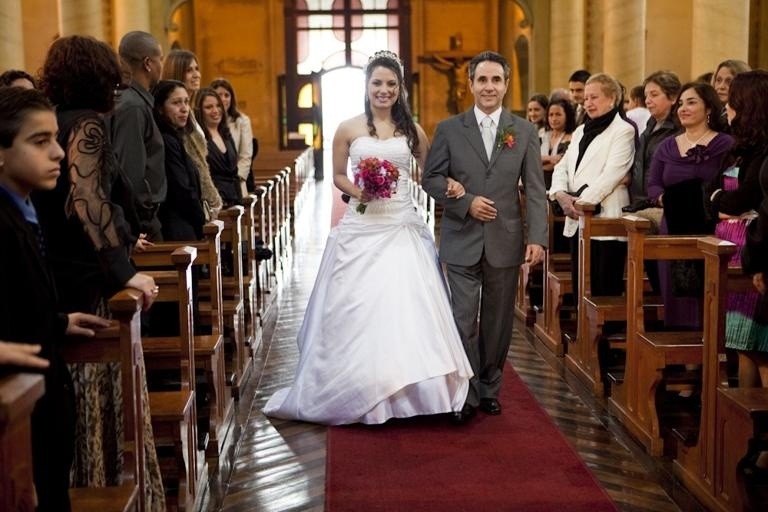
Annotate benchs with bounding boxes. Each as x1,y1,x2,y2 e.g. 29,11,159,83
1,144,315,511
513,194,767,511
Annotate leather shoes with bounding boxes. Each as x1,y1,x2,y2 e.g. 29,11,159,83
449,397,477,425
479,396,502,416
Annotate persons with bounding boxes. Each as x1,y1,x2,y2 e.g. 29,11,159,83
260,49,473,428
420,52,549,425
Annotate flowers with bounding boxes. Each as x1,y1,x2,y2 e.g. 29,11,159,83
496,127,517,149
348,155,401,215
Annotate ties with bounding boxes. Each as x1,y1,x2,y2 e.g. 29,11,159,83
574,107,586,127
481,116,494,164
